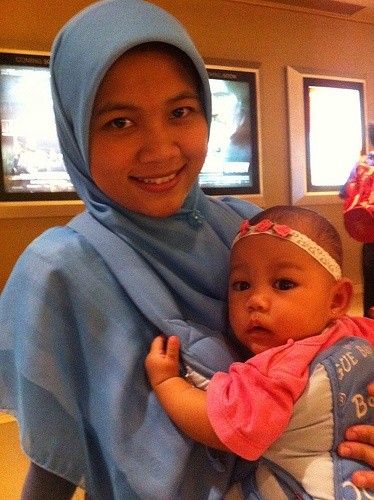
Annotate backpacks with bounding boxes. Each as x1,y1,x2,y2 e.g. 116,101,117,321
342,154,374,243
